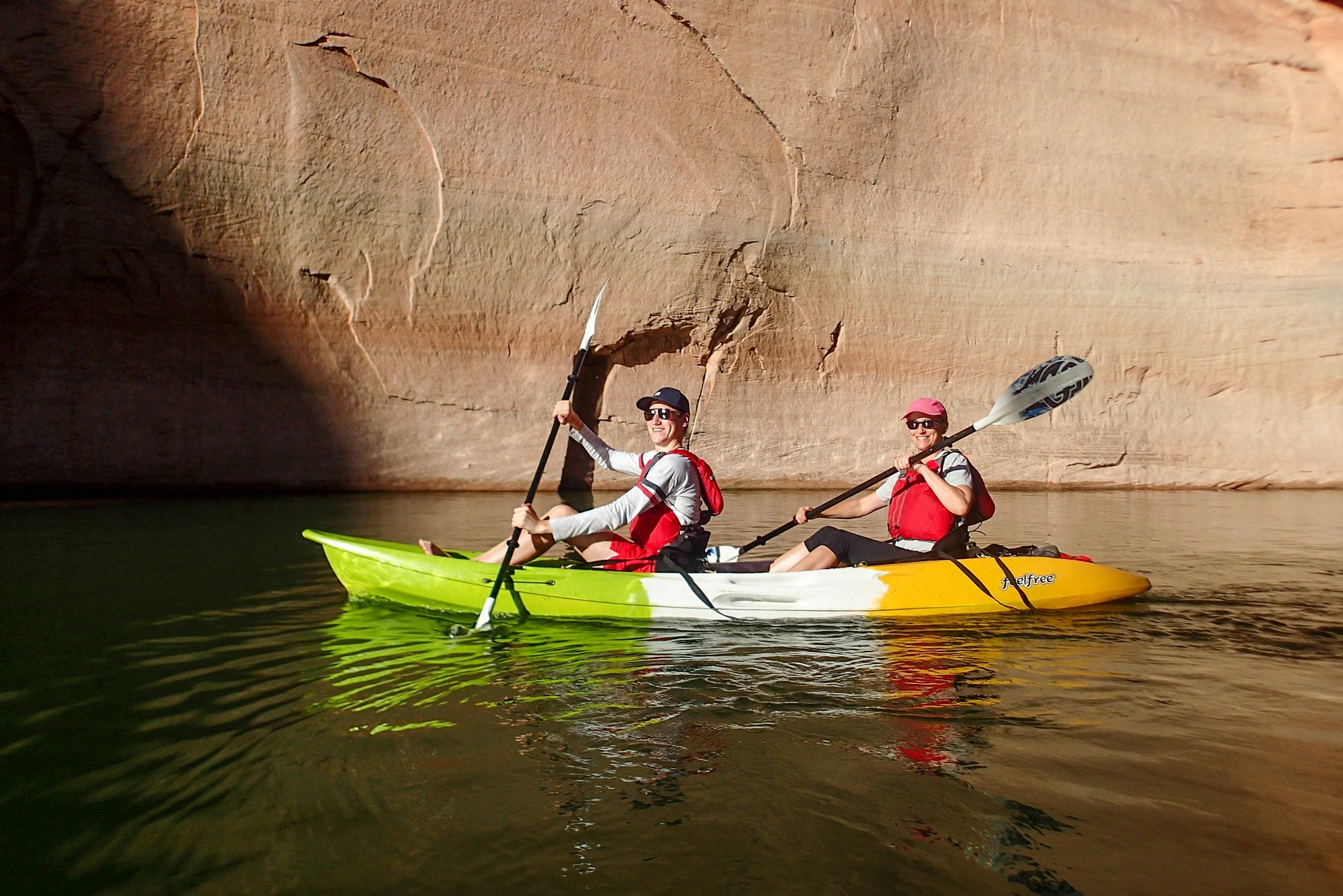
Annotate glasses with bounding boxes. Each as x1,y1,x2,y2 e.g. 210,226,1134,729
906,420,941,430
644,408,687,421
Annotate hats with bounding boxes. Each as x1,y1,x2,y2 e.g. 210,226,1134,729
901,398,948,421
636,387,690,416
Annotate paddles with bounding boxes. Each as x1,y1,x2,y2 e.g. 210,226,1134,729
703,353,1096,560
466,283,614,622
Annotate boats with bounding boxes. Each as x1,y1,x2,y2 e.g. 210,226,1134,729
300,527,1153,622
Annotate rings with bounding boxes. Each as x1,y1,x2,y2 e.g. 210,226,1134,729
903,455,906,457
896,456,899,458
899,456,902,458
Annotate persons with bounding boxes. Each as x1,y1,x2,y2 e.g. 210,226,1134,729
418,387,703,573
756,397,974,573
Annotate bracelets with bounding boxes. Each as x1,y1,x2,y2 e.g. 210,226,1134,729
916,462,922,472
